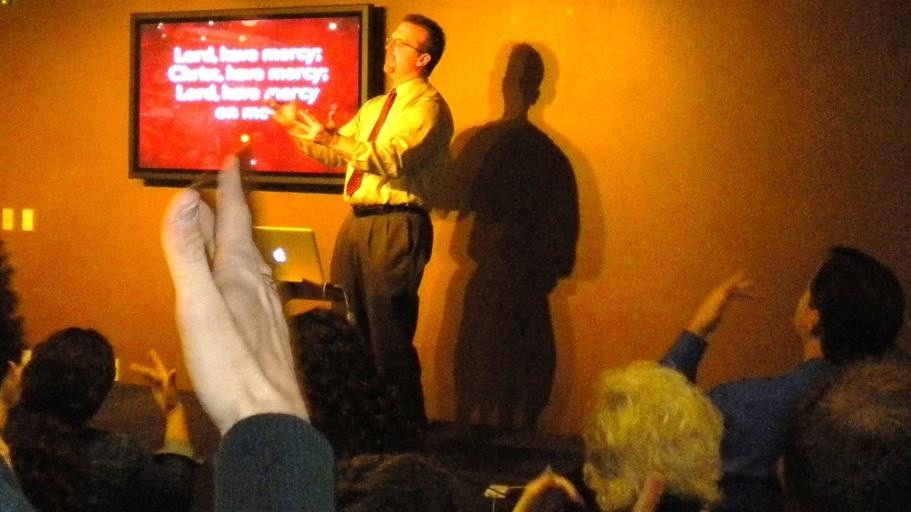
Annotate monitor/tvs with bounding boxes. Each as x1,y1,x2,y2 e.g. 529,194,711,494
127,4,372,185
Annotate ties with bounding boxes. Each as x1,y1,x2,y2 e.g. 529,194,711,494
345,87,399,198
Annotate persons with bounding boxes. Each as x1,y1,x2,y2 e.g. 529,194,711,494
267,15,456,455
0,153,910,512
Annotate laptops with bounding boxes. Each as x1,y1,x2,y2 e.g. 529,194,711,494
252,226,326,287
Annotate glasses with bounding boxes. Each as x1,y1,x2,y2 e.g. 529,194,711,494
385,36,425,55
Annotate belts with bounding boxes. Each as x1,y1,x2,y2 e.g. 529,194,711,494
352,201,430,219
